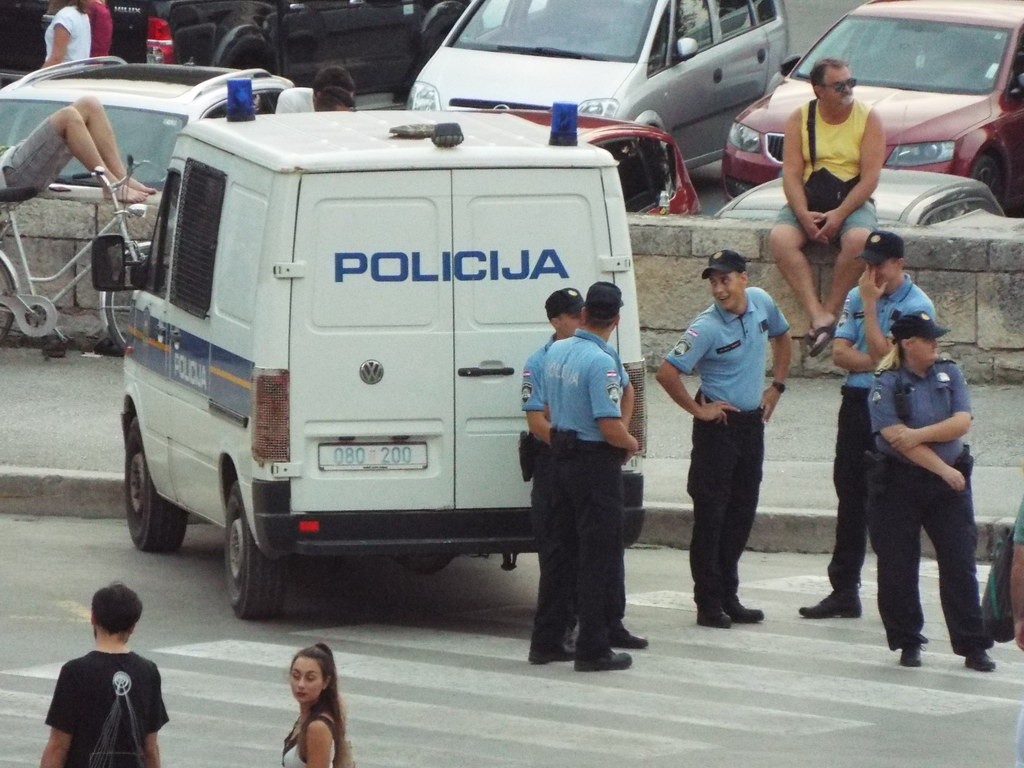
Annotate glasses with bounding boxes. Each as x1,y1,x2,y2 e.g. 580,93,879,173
815,78,857,91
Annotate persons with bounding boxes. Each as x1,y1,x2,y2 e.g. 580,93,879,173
797,230,937,620
765,58,887,357
276,65,357,115
515,282,650,673
281,642,355,767
0,0,113,72
0,94,156,202
1010,495,1024,768
865,310,999,672
38,580,170,768
652,248,793,629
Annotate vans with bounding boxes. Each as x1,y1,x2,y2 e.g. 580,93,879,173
403,0,791,178
89,74,651,624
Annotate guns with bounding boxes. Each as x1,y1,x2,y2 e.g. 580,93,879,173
520,430,531,483
862,449,887,490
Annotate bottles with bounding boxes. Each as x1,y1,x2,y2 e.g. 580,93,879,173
659,191,670,215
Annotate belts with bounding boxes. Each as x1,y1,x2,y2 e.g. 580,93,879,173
575,440,627,455
889,457,938,480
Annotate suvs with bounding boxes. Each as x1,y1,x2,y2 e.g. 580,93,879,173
0,55,297,193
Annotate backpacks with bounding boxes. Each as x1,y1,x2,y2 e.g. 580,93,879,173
981,526,1016,642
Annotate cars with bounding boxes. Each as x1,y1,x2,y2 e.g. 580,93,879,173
0,0,472,101
716,0,1024,220
472,106,705,217
716,167,1007,230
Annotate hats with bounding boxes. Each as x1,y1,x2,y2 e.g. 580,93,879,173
889,310,951,345
701,249,745,279
854,231,904,264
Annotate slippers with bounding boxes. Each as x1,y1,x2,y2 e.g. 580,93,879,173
805,319,838,356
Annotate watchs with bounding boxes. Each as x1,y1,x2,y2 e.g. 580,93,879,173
771,380,786,393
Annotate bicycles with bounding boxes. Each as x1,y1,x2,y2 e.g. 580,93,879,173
0,153,148,357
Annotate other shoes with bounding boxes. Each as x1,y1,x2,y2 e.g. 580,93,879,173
900,644,922,666
799,590,862,618
91,337,123,356
42,334,64,356
529,643,575,663
574,648,632,673
965,649,996,670
727,597,765,620
607,622,648,648
697,606,731,628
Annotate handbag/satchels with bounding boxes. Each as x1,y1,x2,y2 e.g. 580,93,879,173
955,444,973,479
804,167,849,226
